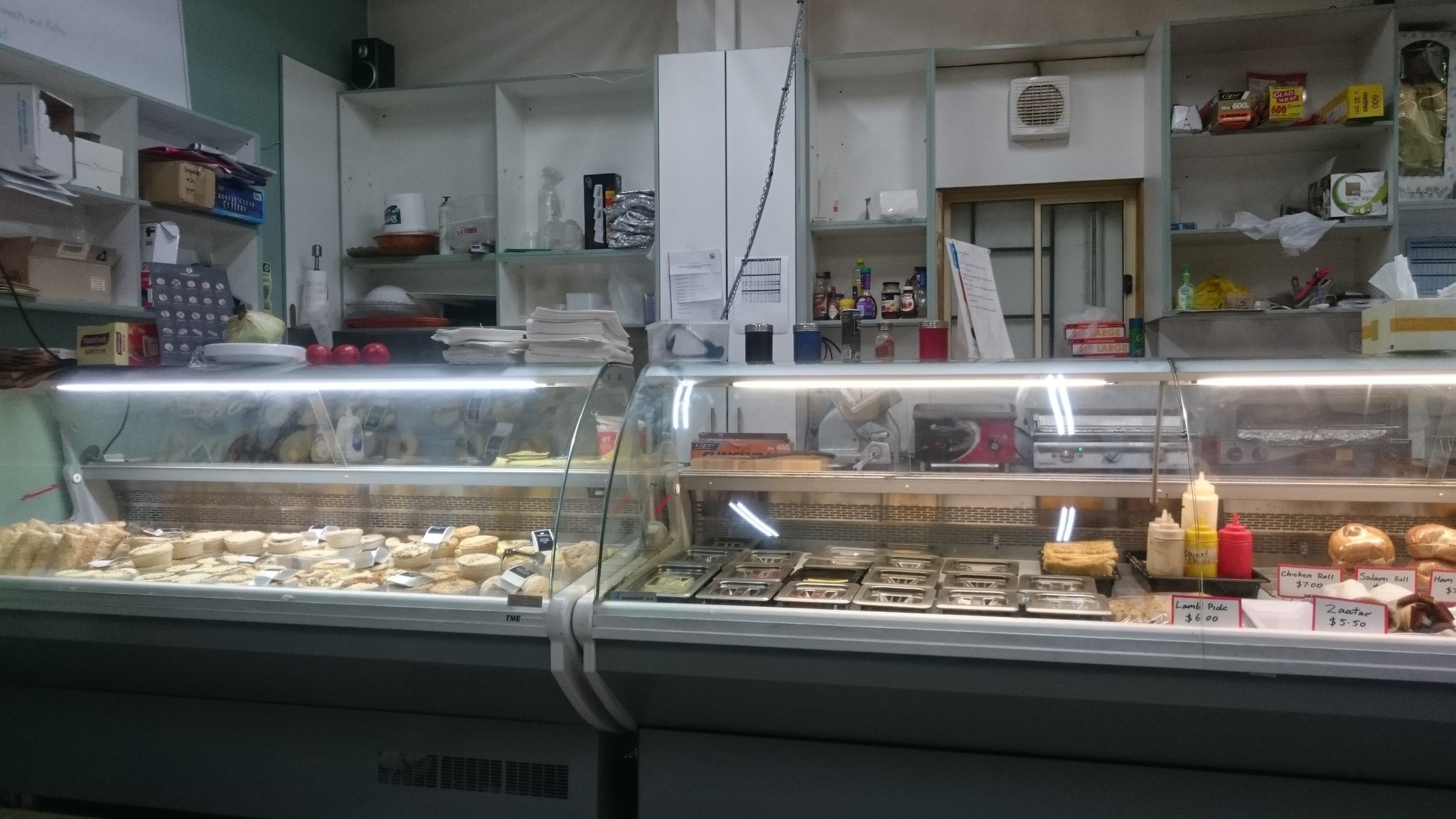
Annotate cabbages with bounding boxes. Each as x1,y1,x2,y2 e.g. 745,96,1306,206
227,309,287,345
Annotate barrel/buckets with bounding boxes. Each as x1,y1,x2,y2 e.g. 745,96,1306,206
383,193,428,235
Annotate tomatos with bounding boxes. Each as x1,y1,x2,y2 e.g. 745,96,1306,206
360,343,391,363
331,345,361,364
305,344,331,364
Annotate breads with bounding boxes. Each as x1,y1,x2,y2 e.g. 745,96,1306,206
1042,540,1118,577
69,524,612,601
0,518,129,576
1327,524,1456,610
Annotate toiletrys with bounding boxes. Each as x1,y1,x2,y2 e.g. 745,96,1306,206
1174,263,1196,311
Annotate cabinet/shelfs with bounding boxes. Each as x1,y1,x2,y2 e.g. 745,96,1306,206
338,67,653,363
653,45,806,362
0,42,264,368
806,0,1456,361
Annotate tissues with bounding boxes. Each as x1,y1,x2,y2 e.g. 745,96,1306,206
1362,253,1456,352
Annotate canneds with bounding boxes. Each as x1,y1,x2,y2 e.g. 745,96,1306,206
839,299,855,320
880,281,901,319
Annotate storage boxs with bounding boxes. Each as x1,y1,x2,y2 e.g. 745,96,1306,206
0,236,121,303
1199,91,1250,133
1314,85,1384,124
1308,157,1388,220
1072,338,1130,358
646,318,729,362
1065,322,1125,339
566,292,604,310
77,323,160,367
444,193,496,248
139,161,264,308
1240,86,1303,130
0,81,124,195
1144,311,1362,358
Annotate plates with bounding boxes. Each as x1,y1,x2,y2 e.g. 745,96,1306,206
346,315,448,328
203,343,306,365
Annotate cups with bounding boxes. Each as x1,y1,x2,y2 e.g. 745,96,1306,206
521,232,538,249
642,293,656,324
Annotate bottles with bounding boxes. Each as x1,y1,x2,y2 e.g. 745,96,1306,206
813,257,927,320
1181,471,1219,529
744,324,774,364
1217,511,1252,579
874,321,895,359
793,324,822,364
1184,516,1218,579
1146,511,1184,578
919,320,948,362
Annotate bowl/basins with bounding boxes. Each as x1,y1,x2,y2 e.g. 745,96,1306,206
372,234,440,256
345,246,379,258
591,409,645,459
1291,285,1327,309
